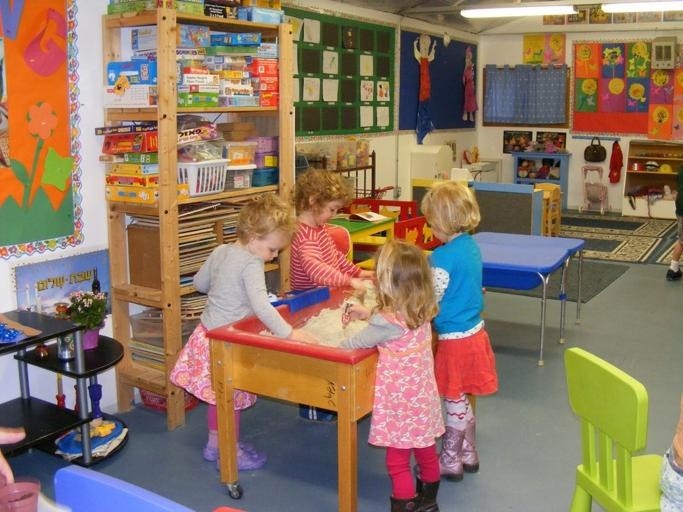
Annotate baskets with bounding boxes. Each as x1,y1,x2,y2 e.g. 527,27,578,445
174,156,231,196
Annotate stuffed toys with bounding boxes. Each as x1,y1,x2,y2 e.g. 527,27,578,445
502,130,566,180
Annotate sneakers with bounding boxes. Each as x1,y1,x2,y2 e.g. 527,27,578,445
216,446,267,469
299,404,333,422
666,268,682,281
204,438,253,461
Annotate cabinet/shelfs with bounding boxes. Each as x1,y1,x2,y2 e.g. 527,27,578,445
623,140,683,221
102,7,297,426
510,152,572,211
295,150,376,209
0,309,129,468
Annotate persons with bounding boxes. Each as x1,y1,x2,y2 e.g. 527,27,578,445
285,165,374,425
664,154,683,283
164,191,300,474
655,394,683,512
329,237,445,512
408,177,503,482
0,429,25,487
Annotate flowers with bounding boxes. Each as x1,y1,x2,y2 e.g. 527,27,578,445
62,290,107,334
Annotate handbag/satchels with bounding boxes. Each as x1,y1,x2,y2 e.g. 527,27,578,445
584,136,607,161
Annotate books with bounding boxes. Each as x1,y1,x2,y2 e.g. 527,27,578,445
124,190,286,377
346,208,384,224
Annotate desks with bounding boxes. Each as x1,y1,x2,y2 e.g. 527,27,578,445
470,230,585,366
543,192,564,235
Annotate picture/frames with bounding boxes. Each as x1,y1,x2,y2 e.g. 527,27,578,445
535,132,567,151
502,131,533,153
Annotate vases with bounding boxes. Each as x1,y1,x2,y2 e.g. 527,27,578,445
80,320,105,350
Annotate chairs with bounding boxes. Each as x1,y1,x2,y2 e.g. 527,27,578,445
546,187,561,236
563,346,665,512
534,183,560,191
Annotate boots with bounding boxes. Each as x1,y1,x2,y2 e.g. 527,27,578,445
388,493,418,512
415,476,439,512
414,427,464,482
460,418,480,473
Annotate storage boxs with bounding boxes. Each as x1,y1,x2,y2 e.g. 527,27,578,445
129,308,201,349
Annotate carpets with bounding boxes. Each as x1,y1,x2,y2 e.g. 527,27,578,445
483,257,631,303
559,209,683,266
